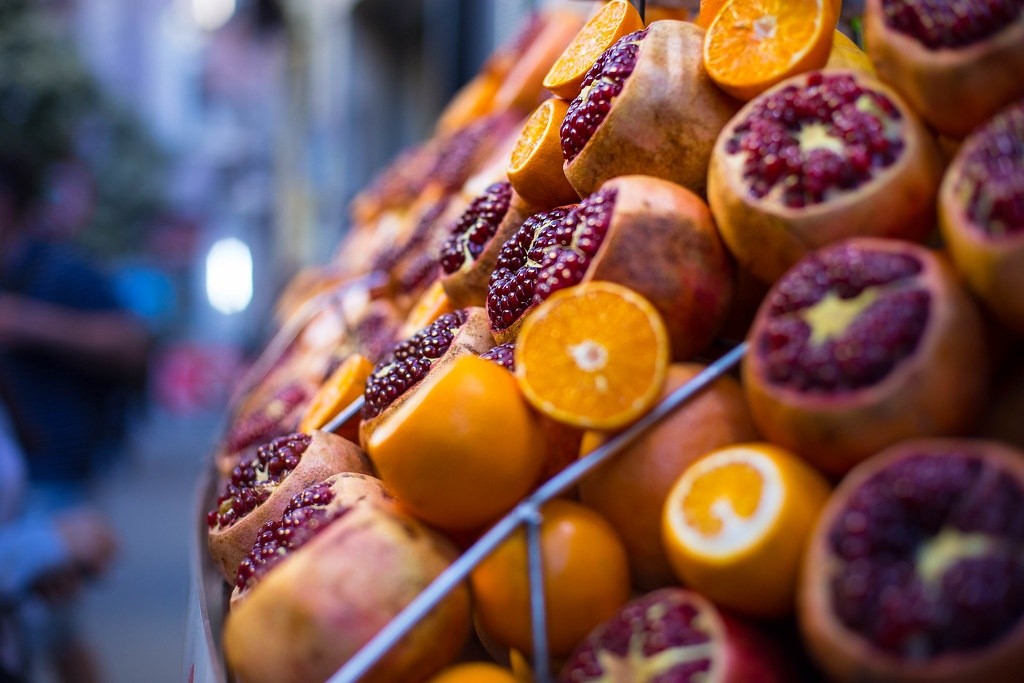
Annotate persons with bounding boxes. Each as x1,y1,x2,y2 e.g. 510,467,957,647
0,0,441,683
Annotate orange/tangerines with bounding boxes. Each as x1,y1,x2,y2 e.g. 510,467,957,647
298,0,881,683
